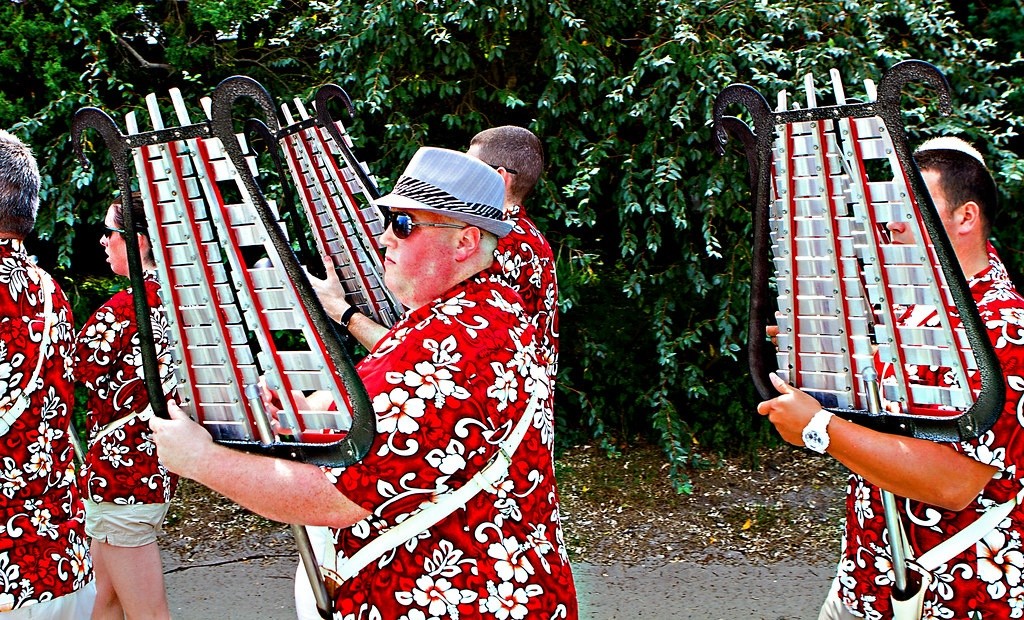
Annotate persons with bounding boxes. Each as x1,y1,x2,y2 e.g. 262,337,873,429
0,130,97,620
758,136,1024,620
149,146,578,620
74,192,181,620
300,125,558,457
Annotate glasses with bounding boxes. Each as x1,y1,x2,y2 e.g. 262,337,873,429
103,224,143,239
382,208,484,240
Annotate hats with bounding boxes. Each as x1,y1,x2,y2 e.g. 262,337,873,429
370,146,516,239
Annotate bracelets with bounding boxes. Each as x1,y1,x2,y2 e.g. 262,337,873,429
342,305,360,330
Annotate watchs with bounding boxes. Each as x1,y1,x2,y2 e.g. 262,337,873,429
801,409,835,455
